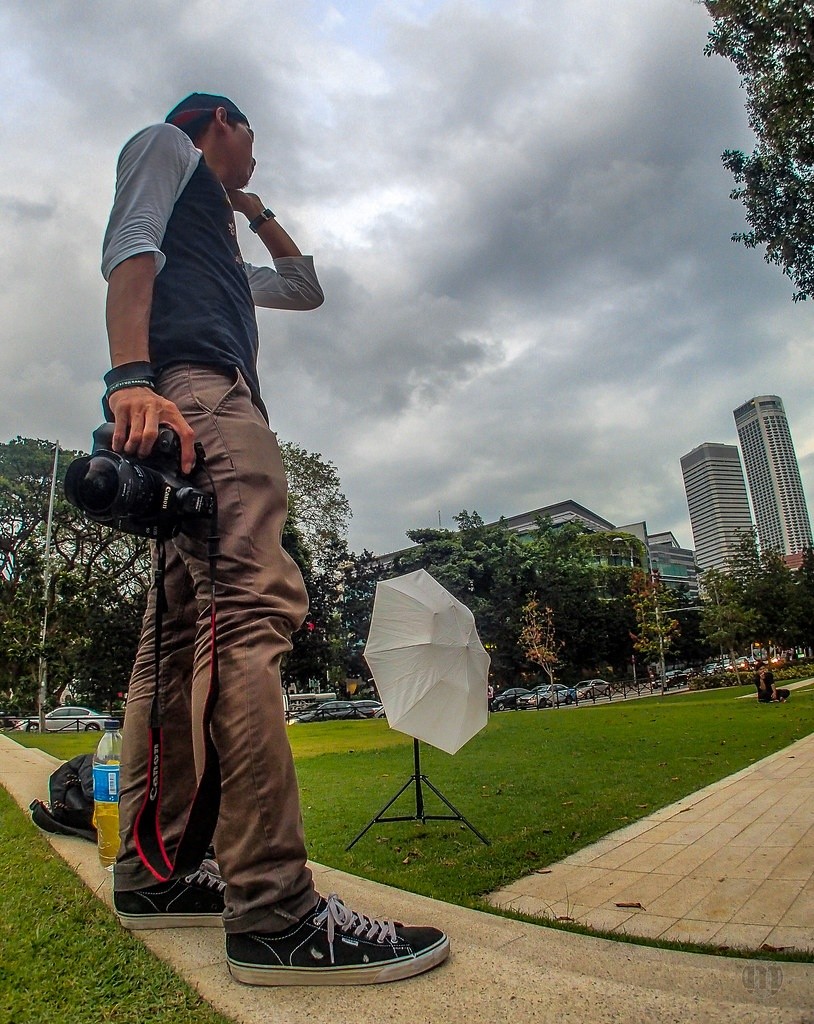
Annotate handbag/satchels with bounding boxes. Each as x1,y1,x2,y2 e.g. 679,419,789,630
28,754,98,843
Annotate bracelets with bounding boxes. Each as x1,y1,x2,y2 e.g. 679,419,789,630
103,360,158,398
247,209,276,234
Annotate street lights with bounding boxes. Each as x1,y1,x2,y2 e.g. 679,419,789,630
613,536,668,691
37,440,61,734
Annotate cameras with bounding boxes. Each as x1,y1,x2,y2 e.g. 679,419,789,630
63,420,216,539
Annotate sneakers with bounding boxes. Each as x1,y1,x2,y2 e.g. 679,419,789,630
113,866,226,930
226,893,450,985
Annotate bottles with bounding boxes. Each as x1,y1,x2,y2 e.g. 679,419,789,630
93,718,135,873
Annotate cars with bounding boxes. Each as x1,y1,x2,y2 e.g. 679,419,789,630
492,687,531,711
18,706,120,732
573,679,613,700
515,684,576,710
652,655,779,690
297,700,386,722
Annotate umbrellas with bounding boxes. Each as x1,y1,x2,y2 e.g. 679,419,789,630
362,569,492,756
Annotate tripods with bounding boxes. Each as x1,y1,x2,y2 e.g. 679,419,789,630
345,739,492,853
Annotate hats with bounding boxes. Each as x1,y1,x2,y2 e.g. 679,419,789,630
165,94,248,128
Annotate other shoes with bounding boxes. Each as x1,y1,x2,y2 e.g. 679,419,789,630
198,845,221,877
780,697,786,703
770,699,779,703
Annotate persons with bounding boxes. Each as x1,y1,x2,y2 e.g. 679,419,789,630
753,660,790,703
101,94,451,987
487,683,493,711
